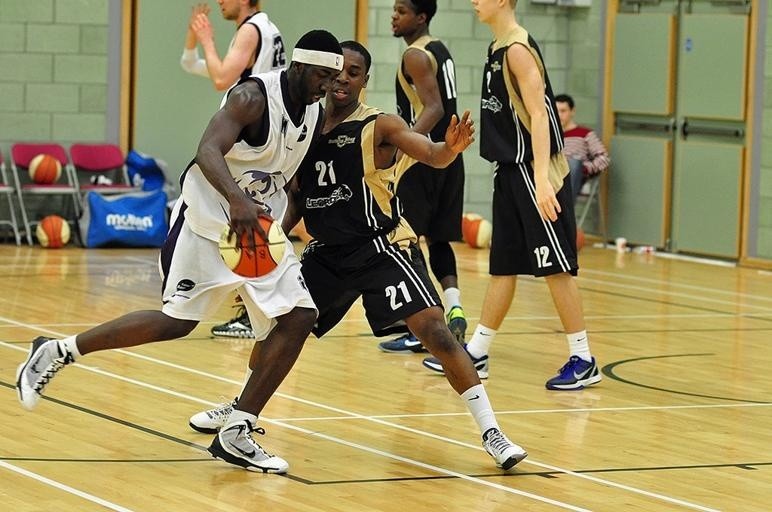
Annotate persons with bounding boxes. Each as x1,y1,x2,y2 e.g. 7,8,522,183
189,39,527,470
550,93,610,205
181,0,294,339
16,29,345,475
424,0,602,392
377,0,466,354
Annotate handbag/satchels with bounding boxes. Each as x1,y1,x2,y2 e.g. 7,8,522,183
74,189,169,249
125,150,181,212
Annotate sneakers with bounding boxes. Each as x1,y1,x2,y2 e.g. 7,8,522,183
380,332,428,354
189,399,239,432
547,357,602,389
207,421,288,476
15,336,75,410
445,306,467,344
210,310,255,338
423,345,491,381
483,428,527,471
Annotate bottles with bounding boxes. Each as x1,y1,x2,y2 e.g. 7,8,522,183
631,246,657,254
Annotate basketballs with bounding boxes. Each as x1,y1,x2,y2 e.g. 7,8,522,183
219,216,284,278
37,216,70,247
29,154,62,185
466,219,492,248
462,213,483,242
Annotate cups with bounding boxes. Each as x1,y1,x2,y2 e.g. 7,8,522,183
616,237,627,254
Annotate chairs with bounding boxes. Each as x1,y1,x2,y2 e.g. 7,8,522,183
575,174,608,247
4,143,80,247
0,156,22,247
62,143,141,222
